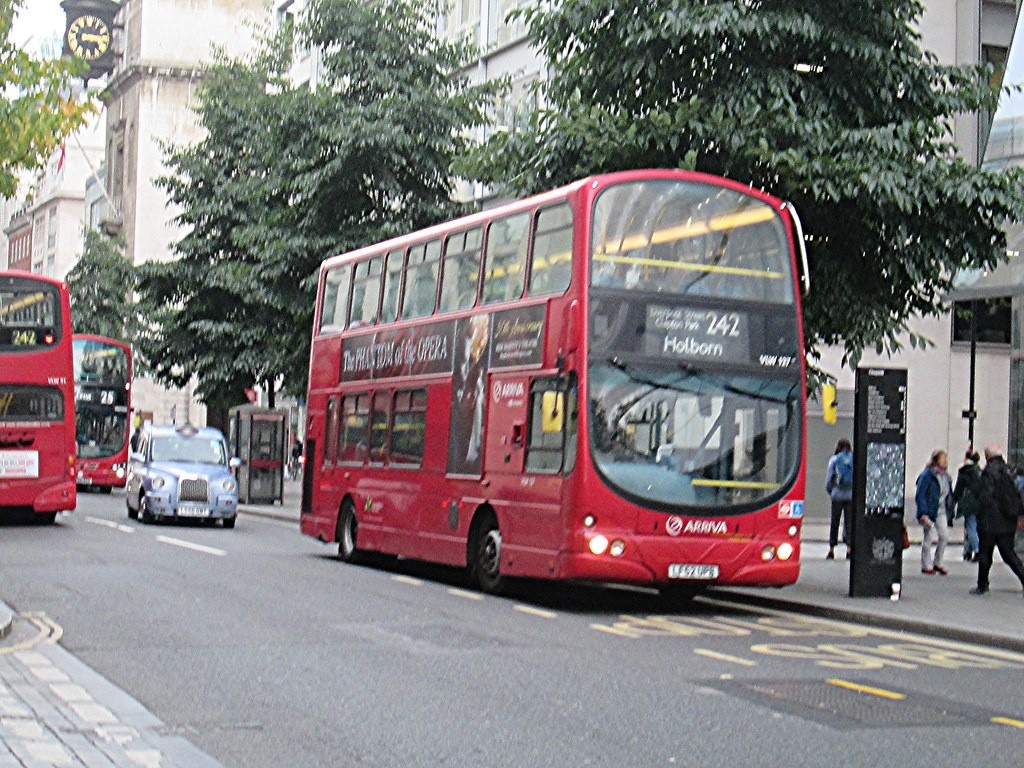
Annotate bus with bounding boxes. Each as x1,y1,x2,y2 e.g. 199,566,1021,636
0,269,77,524
298,168,838,600
67,334,143,496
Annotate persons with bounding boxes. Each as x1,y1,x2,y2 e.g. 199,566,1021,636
954,451,982,563
968,443,1024,595
130,426,140,453
914,447,953,576
291,440,303,458
825,437,854,561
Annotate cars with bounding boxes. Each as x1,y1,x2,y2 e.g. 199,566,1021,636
125,423,249,526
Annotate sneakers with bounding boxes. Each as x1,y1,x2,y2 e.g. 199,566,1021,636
970,588,990,595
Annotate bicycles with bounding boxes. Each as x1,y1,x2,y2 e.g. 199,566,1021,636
288,456,302,481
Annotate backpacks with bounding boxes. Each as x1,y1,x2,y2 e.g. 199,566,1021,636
996,464,1024,520
837,453,852,489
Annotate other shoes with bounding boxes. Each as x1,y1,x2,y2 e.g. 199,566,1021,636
933,565,948,575
922,567,935,575
826,552,835,561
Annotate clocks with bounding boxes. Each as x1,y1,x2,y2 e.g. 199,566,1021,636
67,15,111,60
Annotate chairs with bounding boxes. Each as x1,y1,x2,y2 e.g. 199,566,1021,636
455,258,746,308
354,439,387,462
